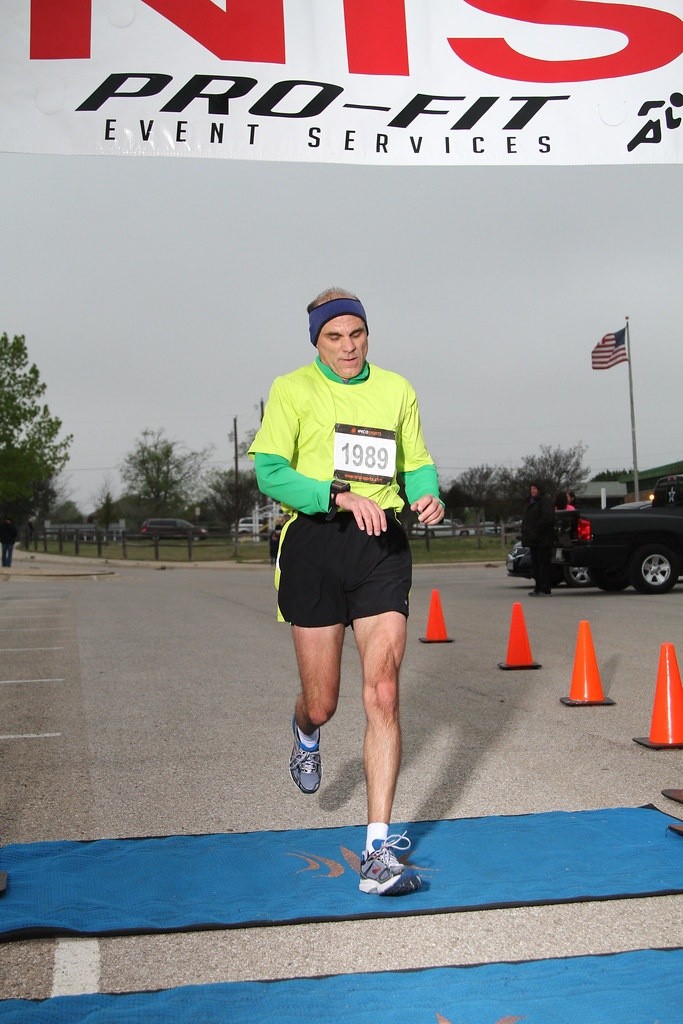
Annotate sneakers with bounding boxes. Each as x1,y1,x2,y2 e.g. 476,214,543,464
358,839,422,896
290,715,322,793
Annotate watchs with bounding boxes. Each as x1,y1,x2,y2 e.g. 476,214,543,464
326,480,351,521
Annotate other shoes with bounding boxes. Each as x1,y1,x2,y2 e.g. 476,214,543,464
527,590,552,597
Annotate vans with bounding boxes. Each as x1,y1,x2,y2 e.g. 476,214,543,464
142,518,207,543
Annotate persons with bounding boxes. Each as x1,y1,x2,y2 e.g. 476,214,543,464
247,288,447,899
521,481,553,597
555,491,574,510
566,491,584,509
0,518,17,566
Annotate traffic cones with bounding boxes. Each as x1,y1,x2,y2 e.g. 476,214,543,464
495,602,542,670
631,643,683,751
558,620,615,707
419,589,454,643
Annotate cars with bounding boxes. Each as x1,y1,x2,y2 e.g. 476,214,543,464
481,522,500,535
507,500,655,587
410,518,474,537
231,516,264,533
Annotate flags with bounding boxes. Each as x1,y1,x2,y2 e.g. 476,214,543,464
591,328,627,370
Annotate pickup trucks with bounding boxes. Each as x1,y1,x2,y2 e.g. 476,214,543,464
548,475,683,594
